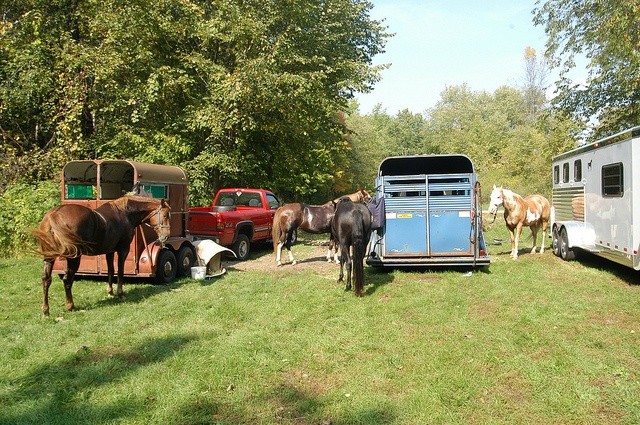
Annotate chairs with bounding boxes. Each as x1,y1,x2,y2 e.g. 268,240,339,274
222,198,234,206
249,199,258,206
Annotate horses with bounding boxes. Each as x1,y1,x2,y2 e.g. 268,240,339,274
331,196,372,298
271,188,372,267
487,184,550,260
19,194,173,317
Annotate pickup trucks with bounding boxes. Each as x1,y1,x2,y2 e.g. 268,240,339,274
188,186,300,259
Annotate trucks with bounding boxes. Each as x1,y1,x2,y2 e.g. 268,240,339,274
549,122,636,274
